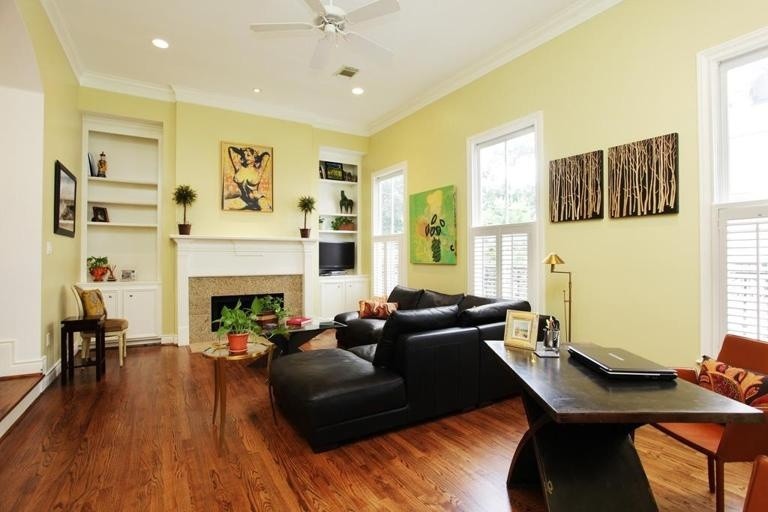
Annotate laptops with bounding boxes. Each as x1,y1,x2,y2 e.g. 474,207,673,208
567,342,679,386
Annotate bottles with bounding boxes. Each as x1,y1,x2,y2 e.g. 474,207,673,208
319,216,324,229
98,151,106,177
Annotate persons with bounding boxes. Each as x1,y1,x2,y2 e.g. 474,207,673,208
224,146,272,211
96,151,108,177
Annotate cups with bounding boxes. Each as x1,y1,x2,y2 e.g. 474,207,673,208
544,331,560,351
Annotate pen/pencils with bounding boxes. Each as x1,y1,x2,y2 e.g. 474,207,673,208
545,315,554,330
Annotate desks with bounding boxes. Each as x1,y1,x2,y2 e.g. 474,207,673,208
482,340,764,512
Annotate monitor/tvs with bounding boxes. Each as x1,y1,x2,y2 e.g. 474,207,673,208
319,242,356,275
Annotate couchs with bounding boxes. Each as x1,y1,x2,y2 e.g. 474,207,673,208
270,285,559,453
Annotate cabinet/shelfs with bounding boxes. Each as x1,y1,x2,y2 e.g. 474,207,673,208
319,145,369,318
81,114,162,350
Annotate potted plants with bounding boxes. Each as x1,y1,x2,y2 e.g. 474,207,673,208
211,299,250,353
331,216,356,231
250,296,282,325
87,256,107,281
171,184,198,235
297,196,316,238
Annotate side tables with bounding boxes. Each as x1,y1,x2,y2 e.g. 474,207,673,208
60,315,106,386
200,341,278,458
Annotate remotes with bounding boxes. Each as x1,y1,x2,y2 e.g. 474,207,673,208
319,321,334,327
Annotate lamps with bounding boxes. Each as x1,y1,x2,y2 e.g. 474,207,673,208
542,254,572,342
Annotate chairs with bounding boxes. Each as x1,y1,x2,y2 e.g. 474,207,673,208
630,334,768,512
743,454,768,512
71,285,128,370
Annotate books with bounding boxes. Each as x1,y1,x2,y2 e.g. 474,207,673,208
286,317,311,325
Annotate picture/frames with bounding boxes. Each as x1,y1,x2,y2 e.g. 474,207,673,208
504,309,540,351
221,141,274,213
325,162,343,180
54,160,77,238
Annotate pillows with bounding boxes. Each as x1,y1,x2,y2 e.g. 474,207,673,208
82,289,107,322
697,356,768,428
358,296,386,319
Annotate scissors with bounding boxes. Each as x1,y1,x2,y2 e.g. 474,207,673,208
552,320,560,330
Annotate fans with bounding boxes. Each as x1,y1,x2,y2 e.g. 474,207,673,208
249,0,401,70
